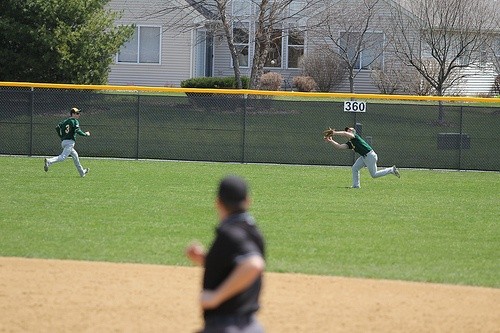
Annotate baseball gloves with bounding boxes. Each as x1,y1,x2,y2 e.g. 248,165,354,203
324,127,333,136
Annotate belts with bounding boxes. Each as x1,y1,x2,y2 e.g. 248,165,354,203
363,150,373,157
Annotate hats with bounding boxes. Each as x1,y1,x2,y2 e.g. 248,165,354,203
71,108,82,113
345,127,356,134
220,176,248,198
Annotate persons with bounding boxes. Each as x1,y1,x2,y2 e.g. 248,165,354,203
44,108,91,178
187,175,267,333
323,126,400,188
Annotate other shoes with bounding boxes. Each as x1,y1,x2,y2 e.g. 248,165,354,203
44,158,50,172
345,185,360,188
393,166,400,178
81,168,89,177
